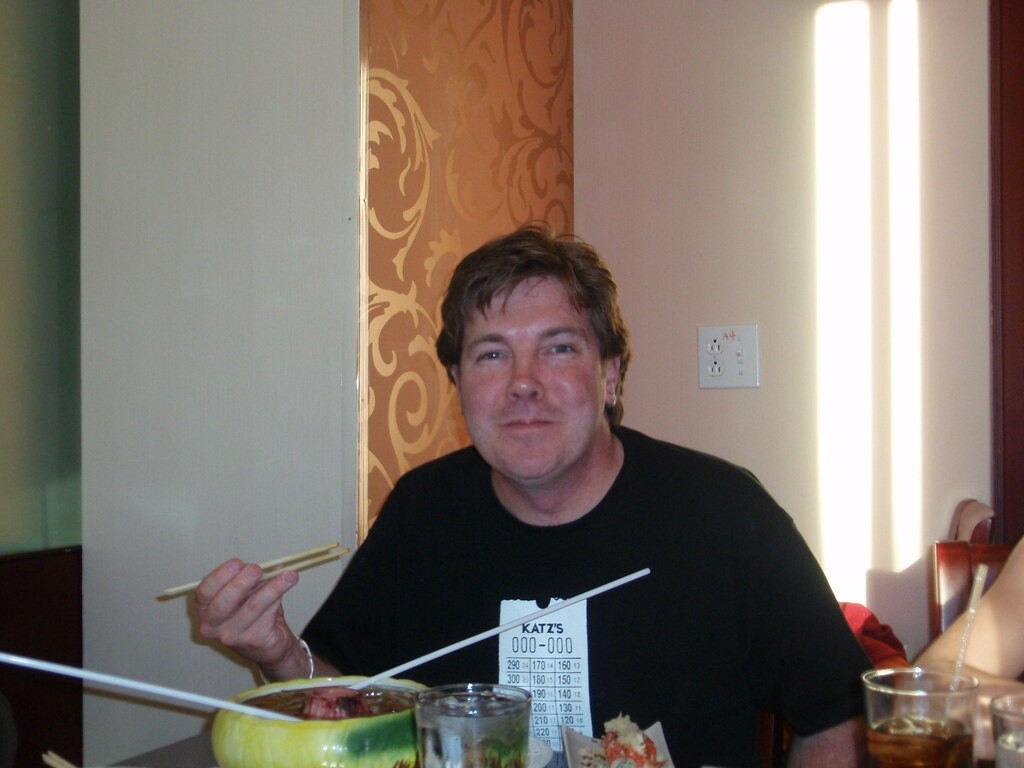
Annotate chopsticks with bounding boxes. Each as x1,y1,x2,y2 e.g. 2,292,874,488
163,543,349,599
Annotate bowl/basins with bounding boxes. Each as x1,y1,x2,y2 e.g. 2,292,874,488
212,676,429,768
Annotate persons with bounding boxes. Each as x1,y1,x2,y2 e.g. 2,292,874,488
196,225,873,768
894,537,1024,736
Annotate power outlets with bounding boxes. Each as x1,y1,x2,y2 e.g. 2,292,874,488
694,322,762,391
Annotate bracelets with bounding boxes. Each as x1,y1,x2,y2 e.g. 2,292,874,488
259,632,314,685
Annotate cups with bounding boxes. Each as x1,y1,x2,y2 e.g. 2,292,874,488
862,666,978,768
416,682,532,768
990,691,1024,768
